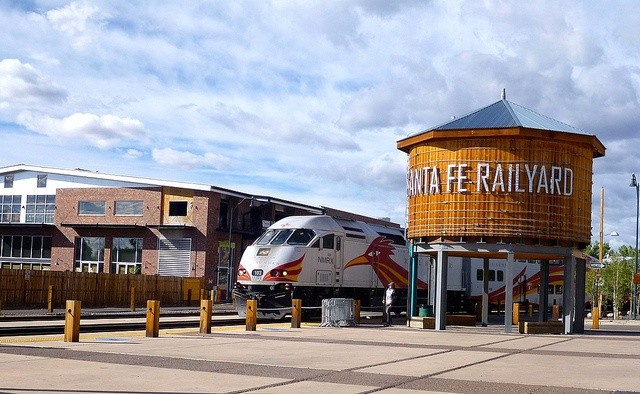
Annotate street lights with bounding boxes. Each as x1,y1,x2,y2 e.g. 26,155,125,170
594,230,620,319
629,173,639,320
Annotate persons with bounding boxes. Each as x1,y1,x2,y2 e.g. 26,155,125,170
382,281,398,327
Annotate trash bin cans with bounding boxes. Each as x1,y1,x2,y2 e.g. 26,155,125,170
418,304,433,317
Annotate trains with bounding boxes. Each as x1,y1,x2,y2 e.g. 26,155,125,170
231,206,605,318
